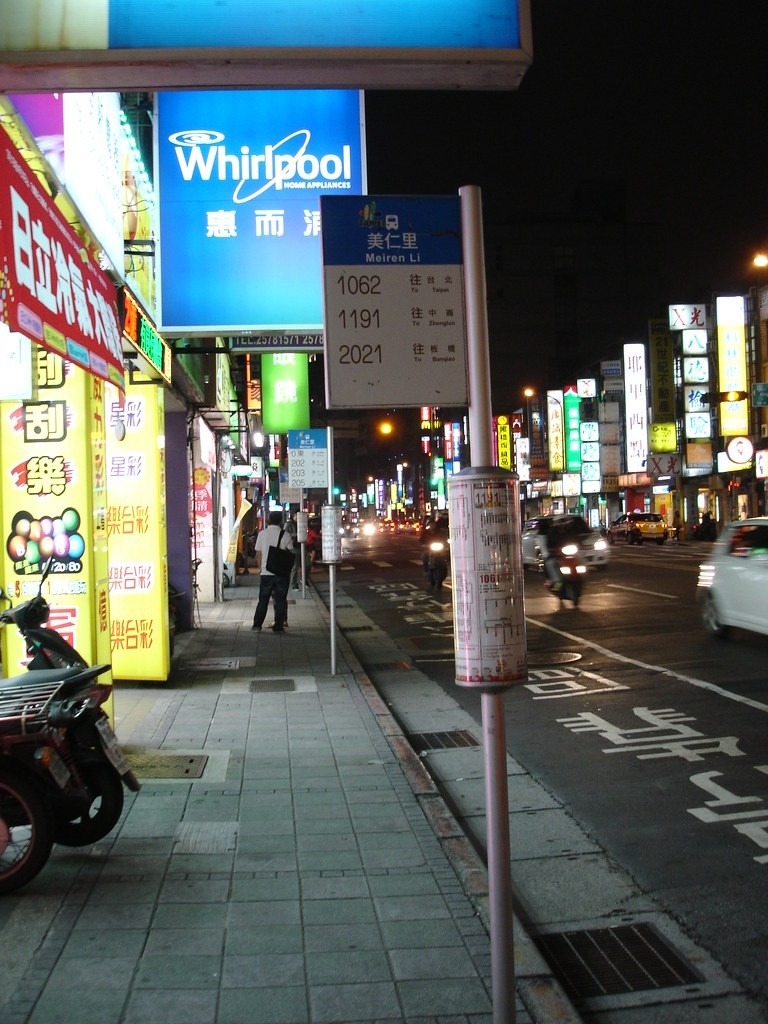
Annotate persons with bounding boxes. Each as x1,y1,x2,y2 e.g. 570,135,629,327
702,511,713,522
281,512,322,591
671,511,683,541
544,515,584,592
251,511,294,634
420,520,448,572
599,519,603,527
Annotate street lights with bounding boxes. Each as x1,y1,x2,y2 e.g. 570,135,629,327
525,390,566,514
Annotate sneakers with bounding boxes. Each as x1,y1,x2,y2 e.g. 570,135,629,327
300,578,310,588
252,623,264,633
291,581,299,591
272,625,285,634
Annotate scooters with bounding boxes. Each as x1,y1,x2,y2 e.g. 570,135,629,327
538,544,587,606
421,540,450,593
1,555,98,686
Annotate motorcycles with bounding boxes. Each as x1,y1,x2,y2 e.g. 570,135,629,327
1,682,92,897
1,662,143,847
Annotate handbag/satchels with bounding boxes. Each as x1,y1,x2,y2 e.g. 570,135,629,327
267,530,296,580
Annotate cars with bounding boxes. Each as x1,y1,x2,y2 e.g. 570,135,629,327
520,514,610,574
379,519,417,535
607,512,668,545
695,518,767,637
